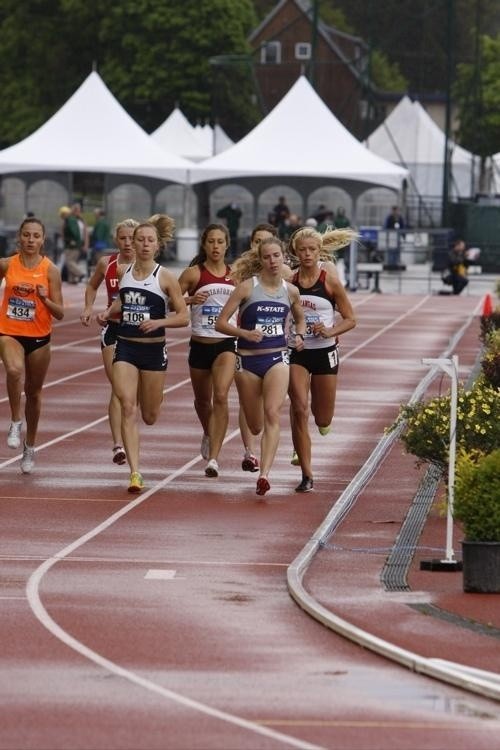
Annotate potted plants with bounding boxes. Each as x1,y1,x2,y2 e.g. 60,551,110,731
436,446,500,594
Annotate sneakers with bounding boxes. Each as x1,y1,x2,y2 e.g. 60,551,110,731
291,451,300,465
319,427,329,435
7,421,22,448
22,439,34,473
242,454,258,472
201,436,210,460
113,446,126,465
256,478,270,495
205,459,218,477
128,474,144,492
295,476,313,492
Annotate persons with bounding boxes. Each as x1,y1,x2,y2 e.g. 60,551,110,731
0,217,64,474
311,204,336,234
61,202,89,282
251,224,277,253
286,228,362,492
289,227,338,465
59,205,85,283
89,209,111,249
80,218,140,465
96,214,189,492
216,201,242,259
272,194,301,237
442,241,469,297
168,225,239,477
382,205,406,266
338,206,351,229
214,238,306,496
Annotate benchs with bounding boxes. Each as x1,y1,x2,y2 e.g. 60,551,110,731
352,263,384,293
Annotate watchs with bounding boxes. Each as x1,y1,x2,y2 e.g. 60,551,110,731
293,333,305,340
